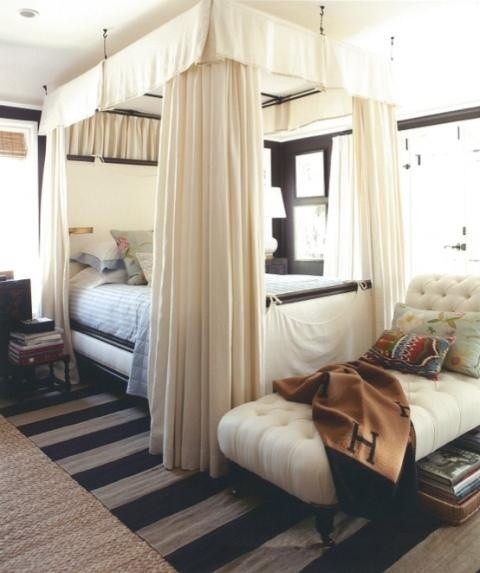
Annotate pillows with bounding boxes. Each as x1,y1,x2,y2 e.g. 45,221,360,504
360,329,457,383
109,229,155,285
70,243,126,273
392,302,480,379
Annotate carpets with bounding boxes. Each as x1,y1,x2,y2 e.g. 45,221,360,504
0,414,176,573
386,512,480,573
0,380,440,573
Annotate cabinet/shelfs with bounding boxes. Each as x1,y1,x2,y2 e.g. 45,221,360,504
0,277,33,387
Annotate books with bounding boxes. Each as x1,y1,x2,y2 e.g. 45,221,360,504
8,317,64,366
416,442,480,503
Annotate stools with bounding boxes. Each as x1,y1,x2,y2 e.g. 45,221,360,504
9,354,71,399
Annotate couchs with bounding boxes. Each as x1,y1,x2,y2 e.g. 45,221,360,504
215,275,480,546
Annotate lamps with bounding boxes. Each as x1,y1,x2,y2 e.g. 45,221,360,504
265,187,288,259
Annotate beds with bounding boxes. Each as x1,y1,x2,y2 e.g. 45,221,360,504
63,225,375,397
